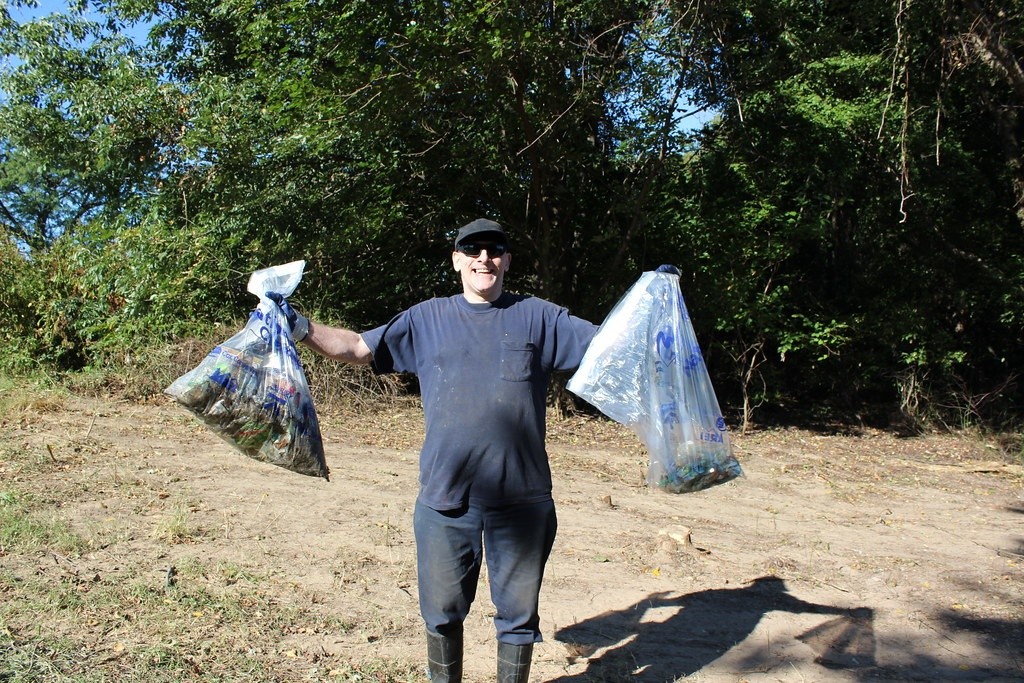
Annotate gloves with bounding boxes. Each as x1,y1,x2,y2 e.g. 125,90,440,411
248,291,310,342
658,265,679,276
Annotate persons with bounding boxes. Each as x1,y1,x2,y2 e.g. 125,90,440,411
265,218,682,683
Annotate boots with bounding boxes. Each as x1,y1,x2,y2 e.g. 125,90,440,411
497,641,533,683
425,627,463,683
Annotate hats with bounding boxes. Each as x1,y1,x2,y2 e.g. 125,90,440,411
455,218,506,252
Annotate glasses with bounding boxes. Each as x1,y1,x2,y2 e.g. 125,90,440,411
456,239,507,257
